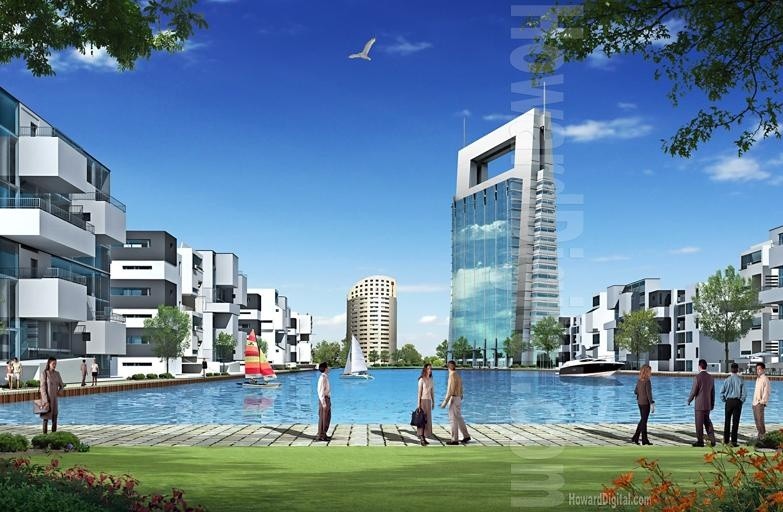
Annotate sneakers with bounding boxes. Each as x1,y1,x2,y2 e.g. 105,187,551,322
420,440,430,446
642,441,654,446
692,442,705,447
631,438,641,445
710,441,717,447
317,436,332,442
461,437,471,443
446,441,459,445
723,440,740,448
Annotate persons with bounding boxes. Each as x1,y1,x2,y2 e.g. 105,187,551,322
40,357,65,434
6,357,23,390
752,362,770,441
80,359,87,386
201,358,208,378
688,360,715,447
263,376,270,384
249,377,256,384
440,361,471,445
418,364,435,446
90,358,99,387
631,365,655,445
317,362,331,441
719,363,746,447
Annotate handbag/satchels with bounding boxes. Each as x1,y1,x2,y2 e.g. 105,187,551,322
410,408,425,430
32,399,51,415
